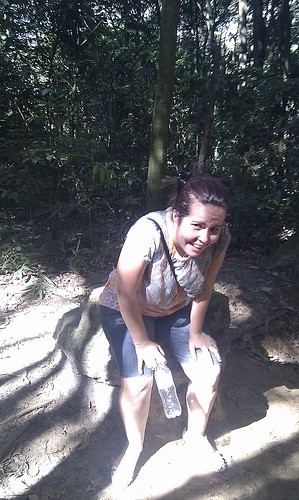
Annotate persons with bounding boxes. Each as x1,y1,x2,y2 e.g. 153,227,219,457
99,175,230,488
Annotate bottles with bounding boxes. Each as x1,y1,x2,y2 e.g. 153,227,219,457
154,360,182,418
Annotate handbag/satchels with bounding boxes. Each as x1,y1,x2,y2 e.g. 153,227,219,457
192,290,231,339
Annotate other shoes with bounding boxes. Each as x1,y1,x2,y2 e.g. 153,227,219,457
110,444,143,487
182,427,226,472
